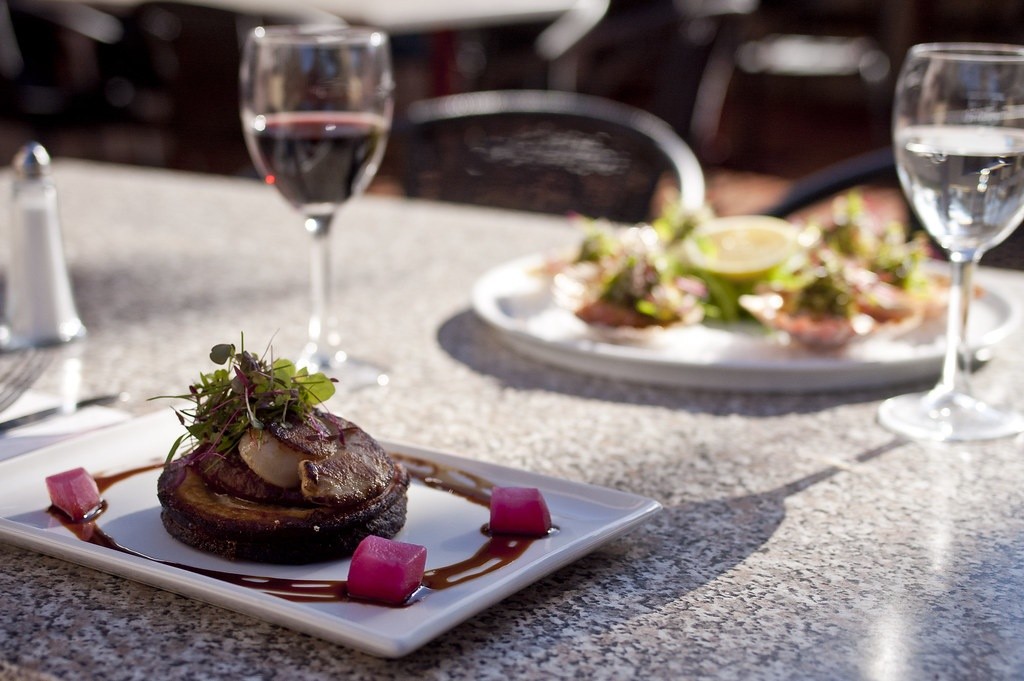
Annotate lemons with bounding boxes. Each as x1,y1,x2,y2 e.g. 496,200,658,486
682,213,802,283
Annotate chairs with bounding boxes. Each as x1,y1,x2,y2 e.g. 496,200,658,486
392,86,704,223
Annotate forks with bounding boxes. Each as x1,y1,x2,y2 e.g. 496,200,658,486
1,343,59,418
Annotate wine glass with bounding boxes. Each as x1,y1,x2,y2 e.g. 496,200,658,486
237,25,396,399
876,37,1023,440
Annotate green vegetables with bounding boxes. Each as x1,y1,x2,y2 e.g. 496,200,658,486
160,331,340,473
573,191,935,326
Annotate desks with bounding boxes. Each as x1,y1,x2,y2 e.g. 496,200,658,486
0,158,1024,681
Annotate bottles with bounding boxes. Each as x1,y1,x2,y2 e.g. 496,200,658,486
8,142,84,344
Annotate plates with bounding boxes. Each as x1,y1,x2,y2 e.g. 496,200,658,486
0,407,662,657
472,232,1014,392
